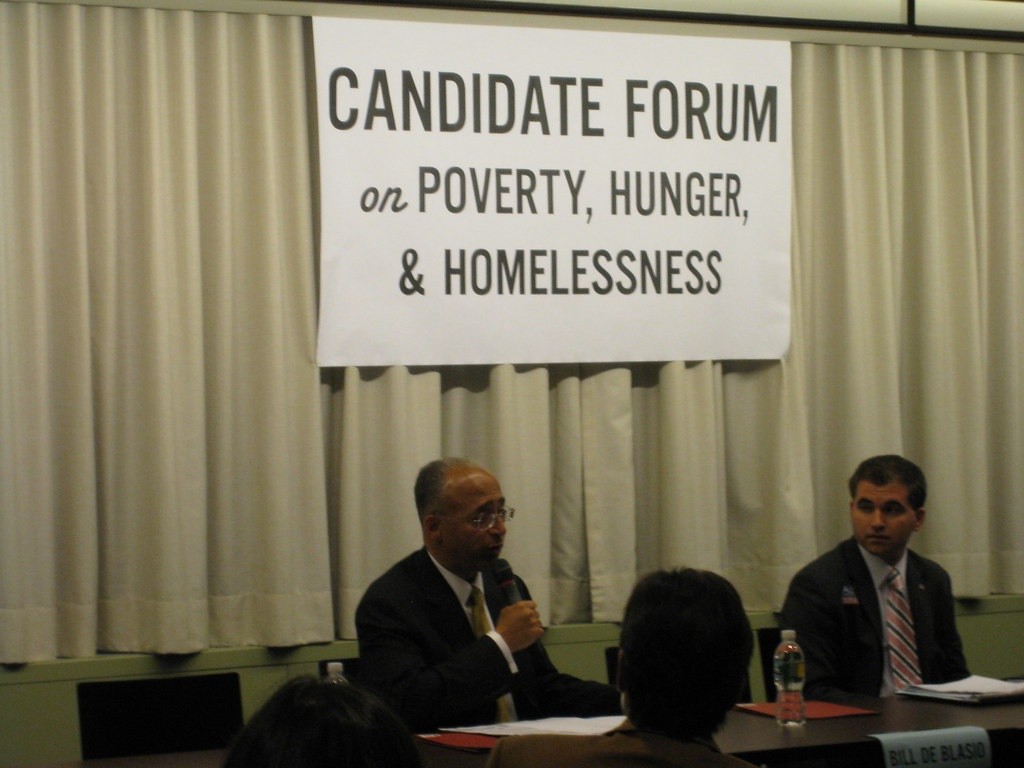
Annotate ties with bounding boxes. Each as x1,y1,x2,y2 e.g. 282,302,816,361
881,565,922,690
466,584,510,726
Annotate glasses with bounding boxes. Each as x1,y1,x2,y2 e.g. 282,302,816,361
436,505,516,531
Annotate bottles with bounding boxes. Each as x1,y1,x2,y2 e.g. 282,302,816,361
773,630,806,726
321,661,349,686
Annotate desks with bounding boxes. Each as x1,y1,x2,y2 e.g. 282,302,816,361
441,677,1024,768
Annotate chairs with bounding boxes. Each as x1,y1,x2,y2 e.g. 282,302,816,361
755,627,783,703
75,669,245,760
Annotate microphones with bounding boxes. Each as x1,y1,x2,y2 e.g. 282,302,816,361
491,559,521,607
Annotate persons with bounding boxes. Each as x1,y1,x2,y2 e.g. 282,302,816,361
215,675,427,768
353,457,622,736
777,454,972,701
484,568,757,768
